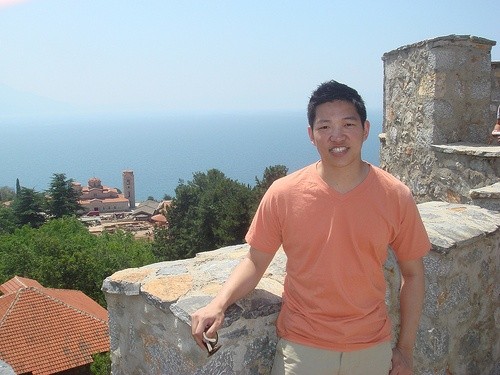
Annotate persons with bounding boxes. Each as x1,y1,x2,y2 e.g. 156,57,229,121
491,104,500,143
191,79,432,375
